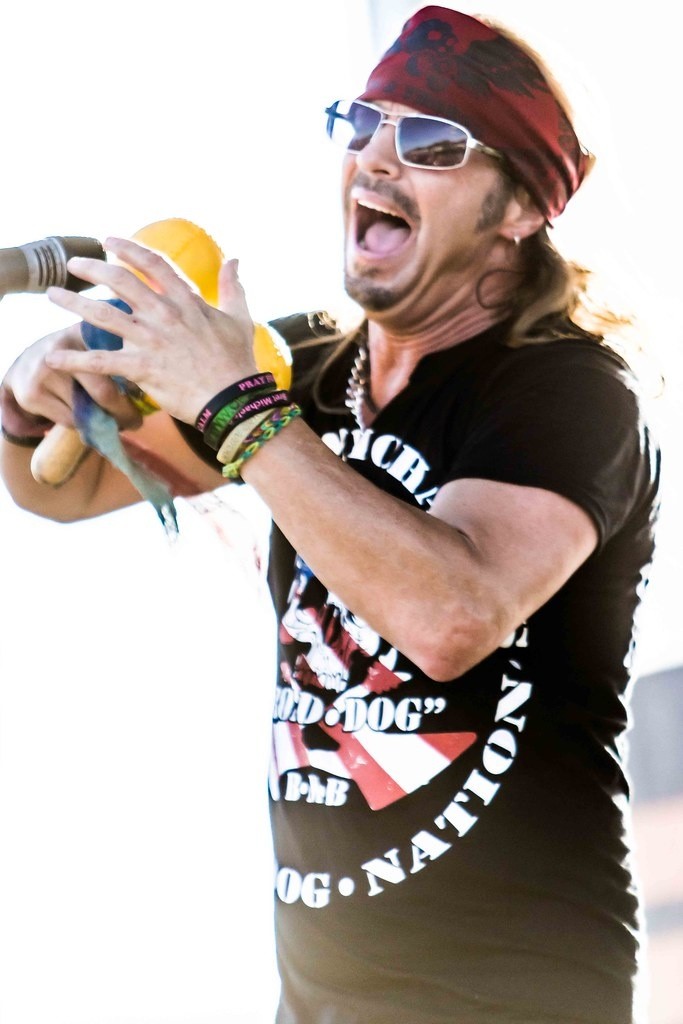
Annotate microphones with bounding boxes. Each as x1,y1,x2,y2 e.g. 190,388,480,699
0,235,106,302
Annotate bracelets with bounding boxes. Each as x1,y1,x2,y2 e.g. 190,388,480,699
0,426,44,447
194,371,303,485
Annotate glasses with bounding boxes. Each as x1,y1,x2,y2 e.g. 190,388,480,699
325,98,505,170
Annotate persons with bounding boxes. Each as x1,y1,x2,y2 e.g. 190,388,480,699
0,6,659,1024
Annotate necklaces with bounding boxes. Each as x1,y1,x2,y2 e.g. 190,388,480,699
345,348,366,434
361,358,380,415
